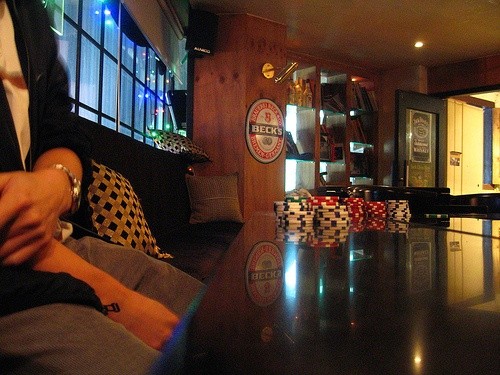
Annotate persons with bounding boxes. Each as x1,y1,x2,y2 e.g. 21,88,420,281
0,0,205,375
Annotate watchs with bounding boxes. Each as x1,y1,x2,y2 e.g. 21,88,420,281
47,163,82,215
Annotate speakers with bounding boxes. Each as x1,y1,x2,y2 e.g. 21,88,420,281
185,9,218,58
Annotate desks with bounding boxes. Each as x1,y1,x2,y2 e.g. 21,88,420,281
142,212,500,375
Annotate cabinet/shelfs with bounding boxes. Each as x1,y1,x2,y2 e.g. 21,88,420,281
285,50,377,188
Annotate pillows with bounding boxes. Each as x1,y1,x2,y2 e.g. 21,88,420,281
148,127,212,163
86,159,172,258
185,173,242,225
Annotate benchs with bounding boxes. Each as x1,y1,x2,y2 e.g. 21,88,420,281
67,112,241,282
